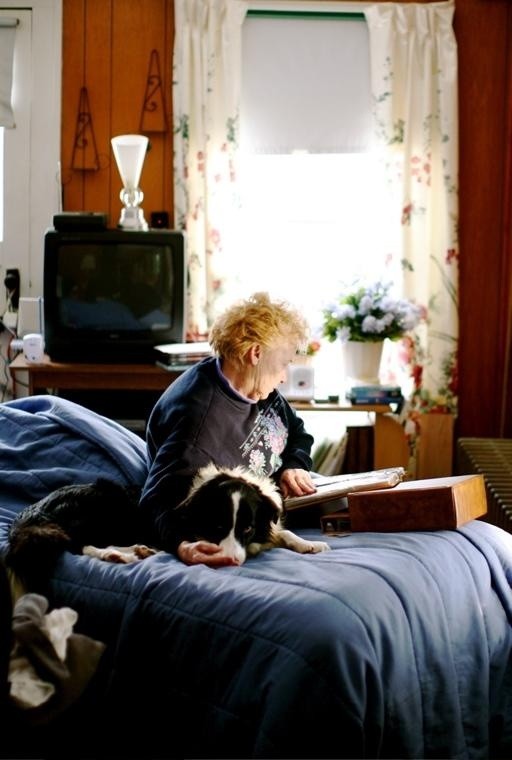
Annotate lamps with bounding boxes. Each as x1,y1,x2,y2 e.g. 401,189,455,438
111,135,150,230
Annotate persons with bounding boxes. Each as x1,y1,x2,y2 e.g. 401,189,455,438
137,289,322,567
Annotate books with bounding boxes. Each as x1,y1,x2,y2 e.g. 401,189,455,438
283,465,406,511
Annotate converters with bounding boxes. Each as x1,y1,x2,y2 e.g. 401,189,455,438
2,310,16,329
5,274,16,289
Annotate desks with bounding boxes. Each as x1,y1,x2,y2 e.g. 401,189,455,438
456,437,512,535
9,353,183,397
290,402,454,481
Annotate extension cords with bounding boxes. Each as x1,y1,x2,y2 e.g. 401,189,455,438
3,268,20,312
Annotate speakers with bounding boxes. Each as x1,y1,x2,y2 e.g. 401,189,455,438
17,296,41,338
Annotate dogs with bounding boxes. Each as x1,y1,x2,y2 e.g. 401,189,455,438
8,462,329,587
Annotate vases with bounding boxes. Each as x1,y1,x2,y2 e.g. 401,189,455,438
344,341,384,378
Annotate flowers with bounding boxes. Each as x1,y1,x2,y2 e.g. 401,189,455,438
320,283,420,344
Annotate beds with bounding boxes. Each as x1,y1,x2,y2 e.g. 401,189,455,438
0,394,512,760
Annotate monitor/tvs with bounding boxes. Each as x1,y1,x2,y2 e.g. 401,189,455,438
43,226,185,365
